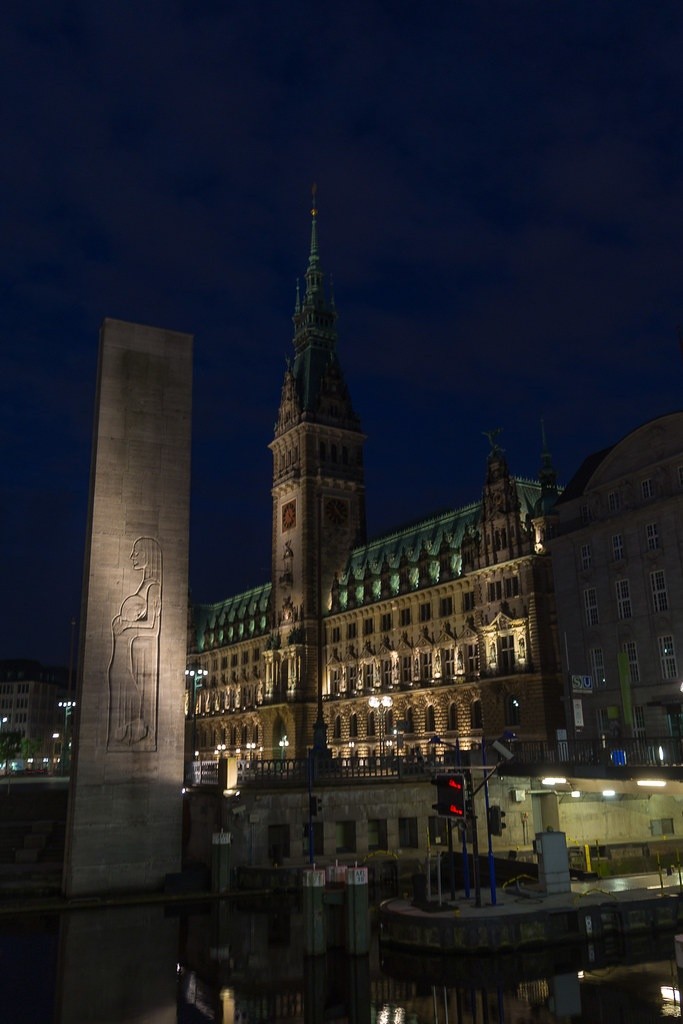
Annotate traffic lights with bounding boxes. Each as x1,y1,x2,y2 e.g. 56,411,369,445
491,805,508,837
434,772,466,820
311,796,324,816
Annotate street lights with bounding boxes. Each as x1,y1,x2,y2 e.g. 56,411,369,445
306,742,327,863
424,734,472,900
367,694,394,756
58,696,78,766
479,731,521,905
187,663,209,752
